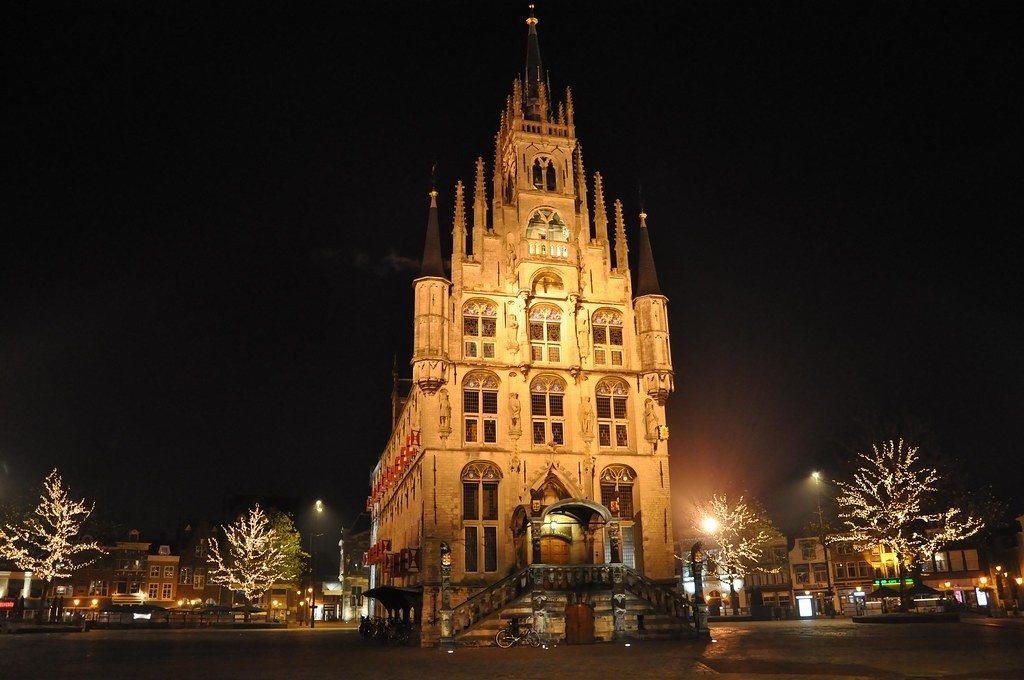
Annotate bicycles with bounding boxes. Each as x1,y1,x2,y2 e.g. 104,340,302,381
495,616,541,649
358,615,413,646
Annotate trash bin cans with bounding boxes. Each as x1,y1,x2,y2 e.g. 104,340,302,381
85,622,91,632
774,608,783,621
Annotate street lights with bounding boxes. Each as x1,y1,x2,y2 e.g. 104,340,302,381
812,471,832,596
310,498,323,626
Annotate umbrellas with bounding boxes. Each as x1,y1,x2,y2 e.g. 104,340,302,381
907,584,942,595
100,605,262,624
867,586,901,613
